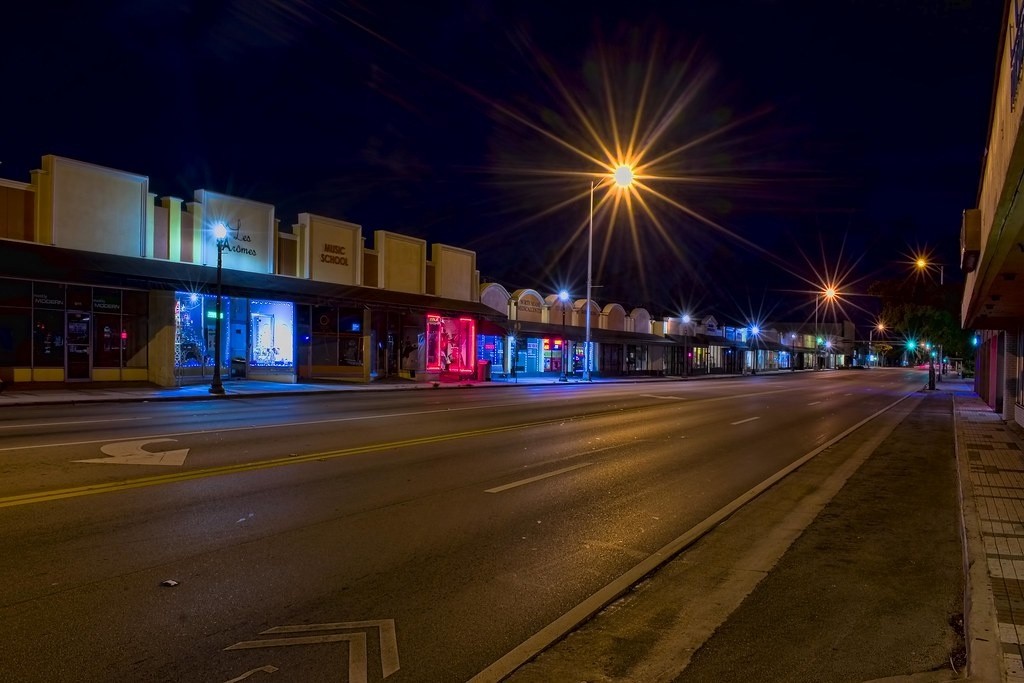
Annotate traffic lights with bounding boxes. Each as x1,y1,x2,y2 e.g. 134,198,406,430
932,351,936,358
909,341,914,350
942,357,946,364
925,342,930,348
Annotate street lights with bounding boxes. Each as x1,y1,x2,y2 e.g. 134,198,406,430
681,314,690,378
916,259,936,391
868,323,875,365
813,286,835,371
209,223,228,393
558,290,569,382
750,326,759,374
582,165,633,380
